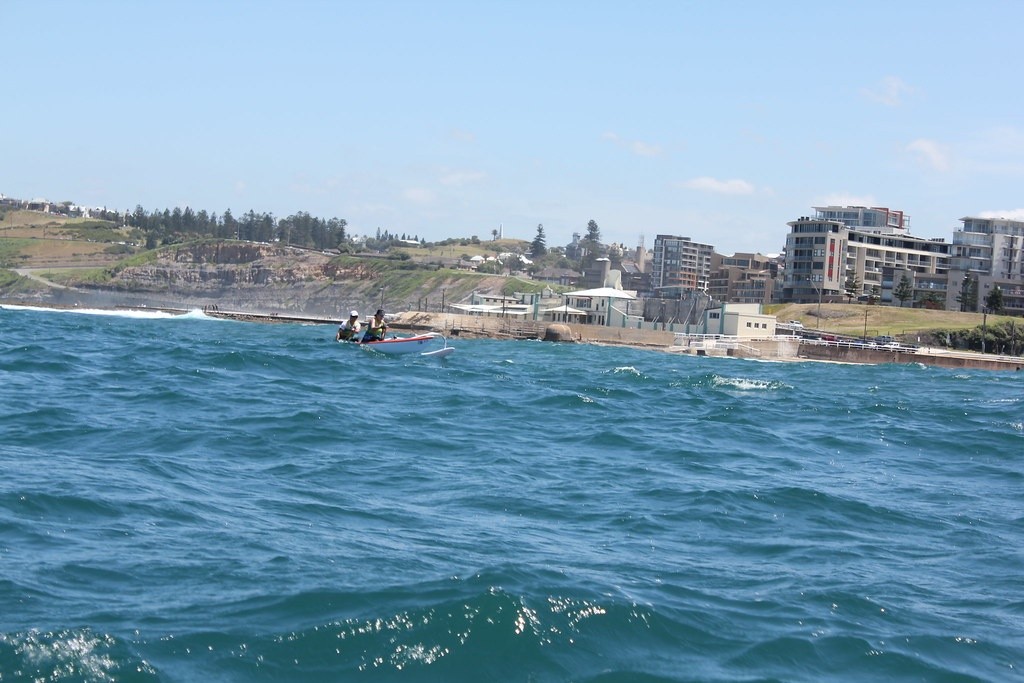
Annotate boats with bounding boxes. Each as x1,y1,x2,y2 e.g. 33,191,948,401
341,333,435,354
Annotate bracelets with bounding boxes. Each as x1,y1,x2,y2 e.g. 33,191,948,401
354,329,355,332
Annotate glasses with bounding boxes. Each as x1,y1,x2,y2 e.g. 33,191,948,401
378,314,385,316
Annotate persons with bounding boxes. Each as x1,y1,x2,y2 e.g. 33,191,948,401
361,309,389,343
408,301,412,311
335,310,361,342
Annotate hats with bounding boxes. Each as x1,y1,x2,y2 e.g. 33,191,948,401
350,310,358,317
